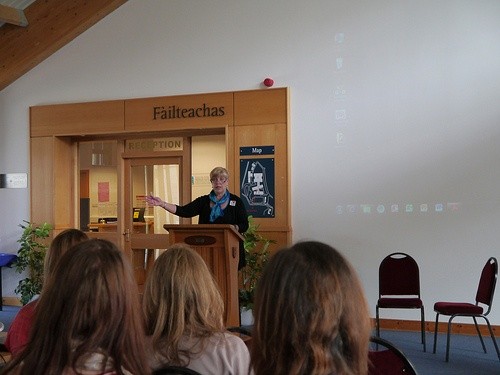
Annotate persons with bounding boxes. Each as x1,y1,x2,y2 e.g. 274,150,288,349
146,167,249,271
4,228,90,361
139,242,255,375
249,242,371,375
0,237,151,375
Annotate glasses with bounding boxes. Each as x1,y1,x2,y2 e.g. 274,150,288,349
211,176,227,182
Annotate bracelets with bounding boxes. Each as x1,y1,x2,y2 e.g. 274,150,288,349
163,202,166,208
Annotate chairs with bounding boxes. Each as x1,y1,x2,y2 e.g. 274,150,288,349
433,257,500,363
376,252,426,352
367,336,418,375
